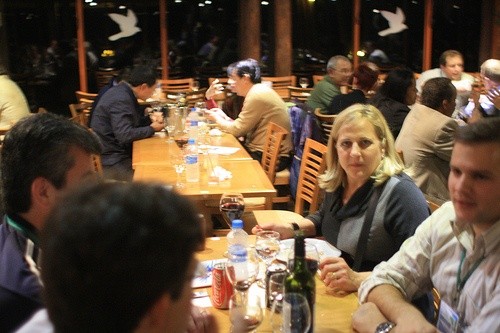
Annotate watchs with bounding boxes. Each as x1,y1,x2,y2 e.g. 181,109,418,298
375,321,396,333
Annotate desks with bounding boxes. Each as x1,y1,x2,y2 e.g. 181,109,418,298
132,131,252,168
191,235,361,333
132,161,278,234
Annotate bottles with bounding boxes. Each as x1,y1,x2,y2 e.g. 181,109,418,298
188,120,200,154
189,105,198,122
183,138,200,182
226,221,250,280
246,280,268,321
229,281,249,324
282,231,318,333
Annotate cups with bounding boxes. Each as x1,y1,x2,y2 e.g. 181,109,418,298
219,193,246,226
299,77,308,89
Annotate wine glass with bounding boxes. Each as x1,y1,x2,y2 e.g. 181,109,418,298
256,231,280,287
160,104,189,189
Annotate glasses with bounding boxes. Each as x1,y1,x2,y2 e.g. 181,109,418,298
331,68,354,74
182,264,209,286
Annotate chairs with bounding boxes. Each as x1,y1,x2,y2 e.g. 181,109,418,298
36,74,448,313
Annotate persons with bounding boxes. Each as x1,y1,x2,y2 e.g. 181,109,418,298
0,66,31,136
14,174,259,333
202,59,293,172
0,112,104,333
303,50,500,207
252,103,435,326
9,22,271,90
87,66,167,183
352,119,500,333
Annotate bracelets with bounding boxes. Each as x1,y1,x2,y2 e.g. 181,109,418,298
291,222,303,236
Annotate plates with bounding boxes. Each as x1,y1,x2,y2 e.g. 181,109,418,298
278,234,342,267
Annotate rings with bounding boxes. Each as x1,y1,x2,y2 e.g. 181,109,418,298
333,272,338,279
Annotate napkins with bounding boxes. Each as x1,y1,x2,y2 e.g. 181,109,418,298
193,258,230,289
209,128,221,137
192,144,240,156
